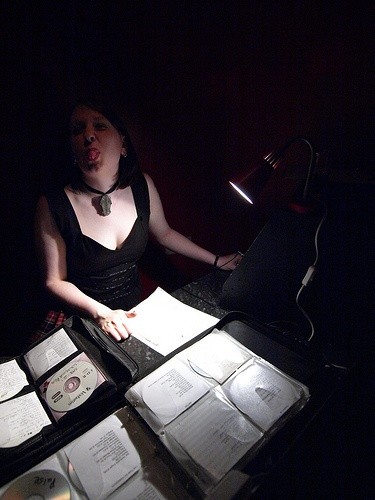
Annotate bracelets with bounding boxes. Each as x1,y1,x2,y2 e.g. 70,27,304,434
214,254,220,267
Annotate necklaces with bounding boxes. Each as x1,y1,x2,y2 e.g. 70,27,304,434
81,170,122,217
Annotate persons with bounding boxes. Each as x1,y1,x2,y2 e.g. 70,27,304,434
33,98,245,341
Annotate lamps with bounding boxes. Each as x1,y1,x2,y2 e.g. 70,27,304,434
229,135,352,214
